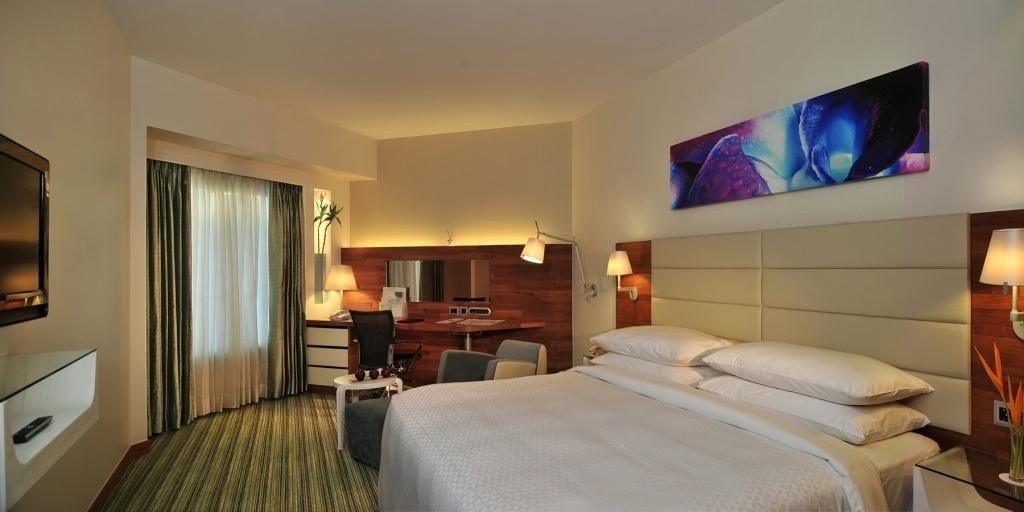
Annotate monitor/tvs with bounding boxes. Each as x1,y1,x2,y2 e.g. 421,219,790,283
0,132,51,327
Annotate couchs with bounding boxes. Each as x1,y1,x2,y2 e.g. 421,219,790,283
439,340,549,399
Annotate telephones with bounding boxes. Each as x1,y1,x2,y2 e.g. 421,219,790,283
329,309,351,321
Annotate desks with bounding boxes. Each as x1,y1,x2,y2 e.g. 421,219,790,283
352,322,545,387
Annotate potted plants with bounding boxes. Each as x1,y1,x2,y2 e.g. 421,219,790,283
314,188,342,305
968,341,1024,488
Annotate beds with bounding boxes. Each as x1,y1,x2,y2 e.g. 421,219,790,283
379,332,943,511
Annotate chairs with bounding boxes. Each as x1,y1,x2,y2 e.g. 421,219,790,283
348,309,423,376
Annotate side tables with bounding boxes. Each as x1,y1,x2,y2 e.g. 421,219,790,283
331,368,400,457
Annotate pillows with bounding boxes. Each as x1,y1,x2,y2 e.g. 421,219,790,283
592,324,733,369
702,341,937,406
589,354,694,385
696,365,939,447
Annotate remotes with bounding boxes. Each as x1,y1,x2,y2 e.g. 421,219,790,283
13,415,53,443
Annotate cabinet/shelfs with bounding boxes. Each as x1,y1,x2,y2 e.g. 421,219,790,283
301,317,348,391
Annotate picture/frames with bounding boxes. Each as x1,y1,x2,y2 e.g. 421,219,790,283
669,62,931,212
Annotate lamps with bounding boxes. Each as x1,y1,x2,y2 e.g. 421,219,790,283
607,250,639,301
979,227,1024,340
326,265,357,310
519,232,596,299
